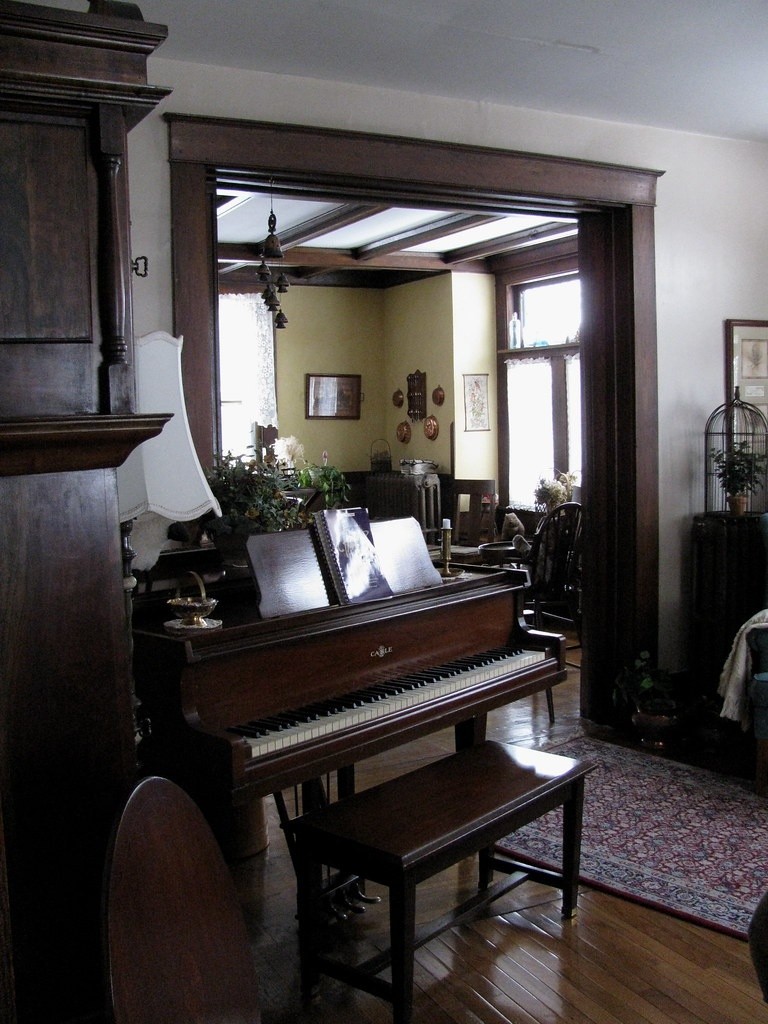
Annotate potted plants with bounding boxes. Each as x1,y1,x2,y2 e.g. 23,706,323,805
611,651,679,747
709,440,768,515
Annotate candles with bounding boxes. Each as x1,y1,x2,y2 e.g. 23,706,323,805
443,518,450,528
459,494,470,513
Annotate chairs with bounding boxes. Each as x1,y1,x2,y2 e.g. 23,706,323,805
499,501,582,668
429,492,499,567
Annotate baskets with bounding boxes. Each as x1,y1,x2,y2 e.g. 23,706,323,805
370,439,391,472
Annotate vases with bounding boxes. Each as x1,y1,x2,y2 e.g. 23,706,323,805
212,531,251,568
309,469,326,486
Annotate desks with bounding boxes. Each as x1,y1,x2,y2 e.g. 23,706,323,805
477,540,535,570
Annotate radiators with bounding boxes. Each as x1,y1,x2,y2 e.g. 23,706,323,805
338,470,442,545
688,511,768,698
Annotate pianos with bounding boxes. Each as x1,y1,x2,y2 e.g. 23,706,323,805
132,556,569,920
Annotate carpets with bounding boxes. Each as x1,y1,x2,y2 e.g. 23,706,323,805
493,735,768,943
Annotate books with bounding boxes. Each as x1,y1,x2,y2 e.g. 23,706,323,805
310,507,393,606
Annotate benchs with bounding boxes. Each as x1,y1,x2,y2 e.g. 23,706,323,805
279,739,599,1024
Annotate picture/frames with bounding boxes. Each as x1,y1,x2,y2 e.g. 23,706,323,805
305,373,362,420
724,319,768,460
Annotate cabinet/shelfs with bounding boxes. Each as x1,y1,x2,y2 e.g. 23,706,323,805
0,0,173,1024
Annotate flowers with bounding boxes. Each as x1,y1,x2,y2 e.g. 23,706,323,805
534,471,574,519
296,449,352,510
166,435,306,548
